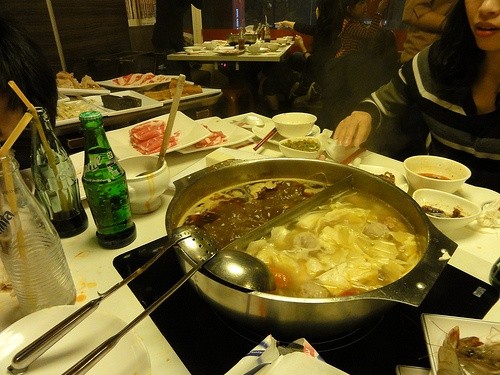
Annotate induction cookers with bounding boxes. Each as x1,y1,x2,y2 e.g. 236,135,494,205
111,235,500,375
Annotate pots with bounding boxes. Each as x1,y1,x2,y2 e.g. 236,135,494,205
163,156,458,327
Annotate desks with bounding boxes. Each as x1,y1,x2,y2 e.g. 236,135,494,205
54,89,224,149
167,42,295,116
0,111,500,375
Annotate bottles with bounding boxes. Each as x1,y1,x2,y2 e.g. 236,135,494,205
238,28,244,50
0,150,77,316
78,109,137,248
27,107,88,239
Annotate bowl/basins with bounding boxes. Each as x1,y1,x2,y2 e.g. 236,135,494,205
272,112,317,139
204,41,217,50
116,155,169,213
247,45,260,54
403,155,472,193
278,136,322,160
268,35,293,52
412,187,481,231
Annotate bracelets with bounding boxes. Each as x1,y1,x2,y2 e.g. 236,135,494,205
303,51,309,58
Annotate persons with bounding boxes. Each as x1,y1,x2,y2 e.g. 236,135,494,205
275,0,500,193
0,16,58,143
152,0,204,80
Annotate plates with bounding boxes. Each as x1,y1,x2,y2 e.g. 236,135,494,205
421,312,500,375
185,44,286,55
252,121,320,144
358,164,409,193
54,73,274,200
0,304,153,375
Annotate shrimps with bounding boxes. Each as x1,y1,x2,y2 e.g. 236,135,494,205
436,326,500,375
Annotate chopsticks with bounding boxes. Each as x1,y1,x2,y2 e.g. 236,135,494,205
253,127,278,151
